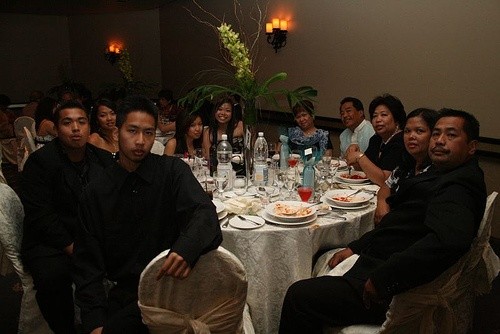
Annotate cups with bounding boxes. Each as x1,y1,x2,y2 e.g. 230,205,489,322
298,186,313,202
322,157,331,166
213,171,236,187
232,176,248,197
193,148,205,158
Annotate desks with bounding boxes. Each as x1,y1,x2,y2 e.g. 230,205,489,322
180,154,382,334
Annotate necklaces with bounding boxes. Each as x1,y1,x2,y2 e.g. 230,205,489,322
381,130,403,145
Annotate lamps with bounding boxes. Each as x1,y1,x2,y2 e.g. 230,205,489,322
104,45,120,63
266,18,288,54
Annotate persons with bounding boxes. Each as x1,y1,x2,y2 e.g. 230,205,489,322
0,83,486,334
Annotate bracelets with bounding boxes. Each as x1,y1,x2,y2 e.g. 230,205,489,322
357,152,364,162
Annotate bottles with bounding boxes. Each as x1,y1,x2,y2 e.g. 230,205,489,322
303,154,315,202
253,132,268,186
316,176,331,195
209,131,218,176
280,138,289,171
216,135,232,189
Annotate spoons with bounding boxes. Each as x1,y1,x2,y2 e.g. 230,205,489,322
259,187,271,197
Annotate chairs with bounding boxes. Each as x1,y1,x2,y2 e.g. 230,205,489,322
13,116,36,172
24,127,37,154
331,191,499,334
151,140,164,155
138,244,257,334
1,183,53,334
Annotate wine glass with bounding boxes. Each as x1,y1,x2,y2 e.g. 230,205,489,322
283,170,300,200
215,178,228,198
287,159,299,168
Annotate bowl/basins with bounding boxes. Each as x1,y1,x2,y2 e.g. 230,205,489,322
266,201,316,221
325,189,375,206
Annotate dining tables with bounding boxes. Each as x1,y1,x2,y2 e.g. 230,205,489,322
155,127,176,145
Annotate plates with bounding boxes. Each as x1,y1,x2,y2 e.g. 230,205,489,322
248,187,279,197
318,204,332,215
335,171,368,184
212,201,228,227
262,209,317,225
319,160,347,169
229,215,265,230
320,196,370,209
